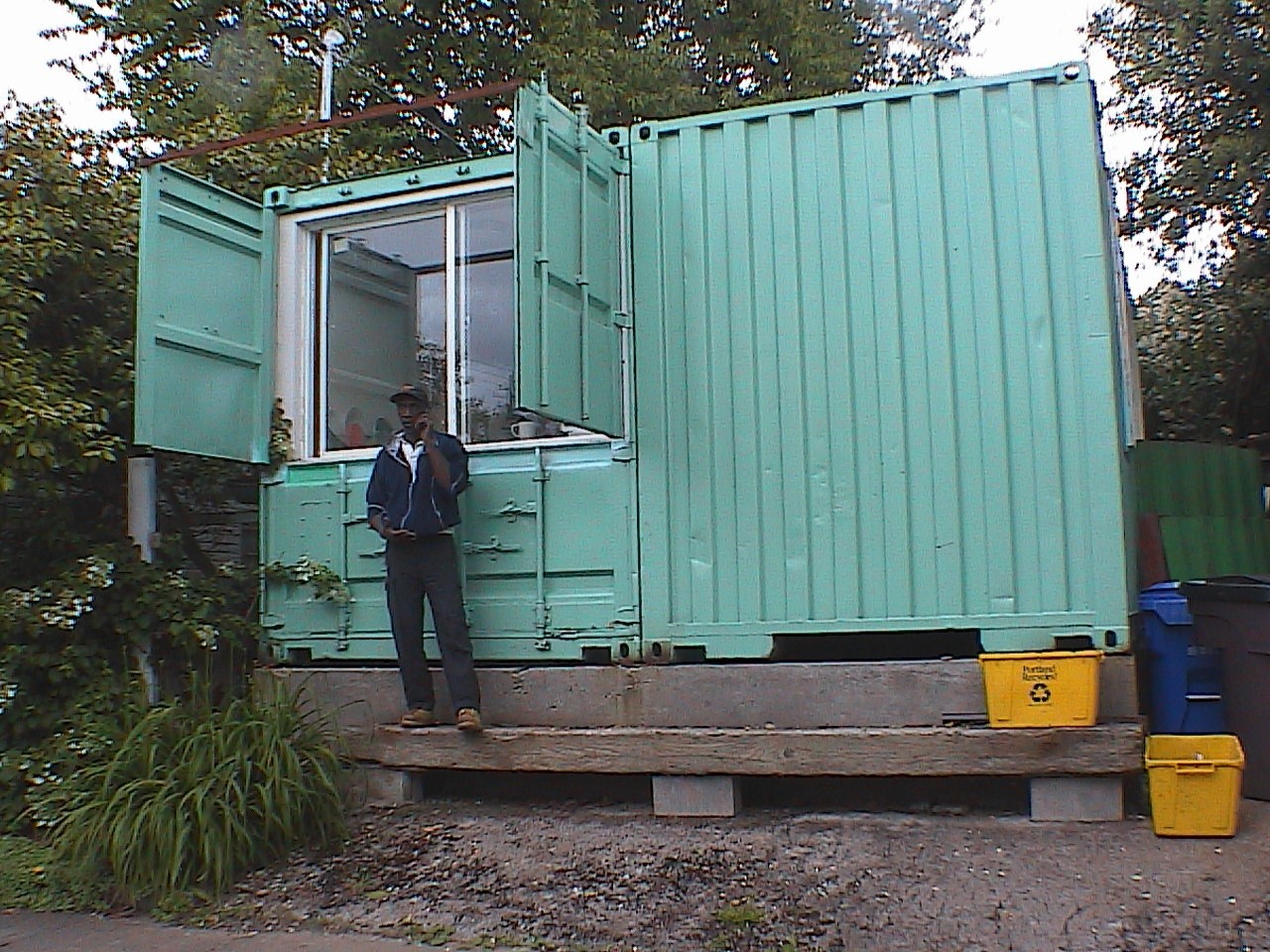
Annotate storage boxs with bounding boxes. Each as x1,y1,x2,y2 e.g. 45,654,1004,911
979,650,1107,728
1144,735,1246,839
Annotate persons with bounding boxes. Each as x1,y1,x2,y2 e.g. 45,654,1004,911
366,383,482,730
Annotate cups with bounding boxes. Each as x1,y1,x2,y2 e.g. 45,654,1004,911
510,421,537,439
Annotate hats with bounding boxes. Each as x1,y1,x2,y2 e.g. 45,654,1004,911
389,383,429,406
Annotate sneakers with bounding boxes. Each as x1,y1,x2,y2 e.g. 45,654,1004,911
400,709,436,727
457,708,483,732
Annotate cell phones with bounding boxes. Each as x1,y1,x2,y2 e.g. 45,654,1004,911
418,409,429,430
